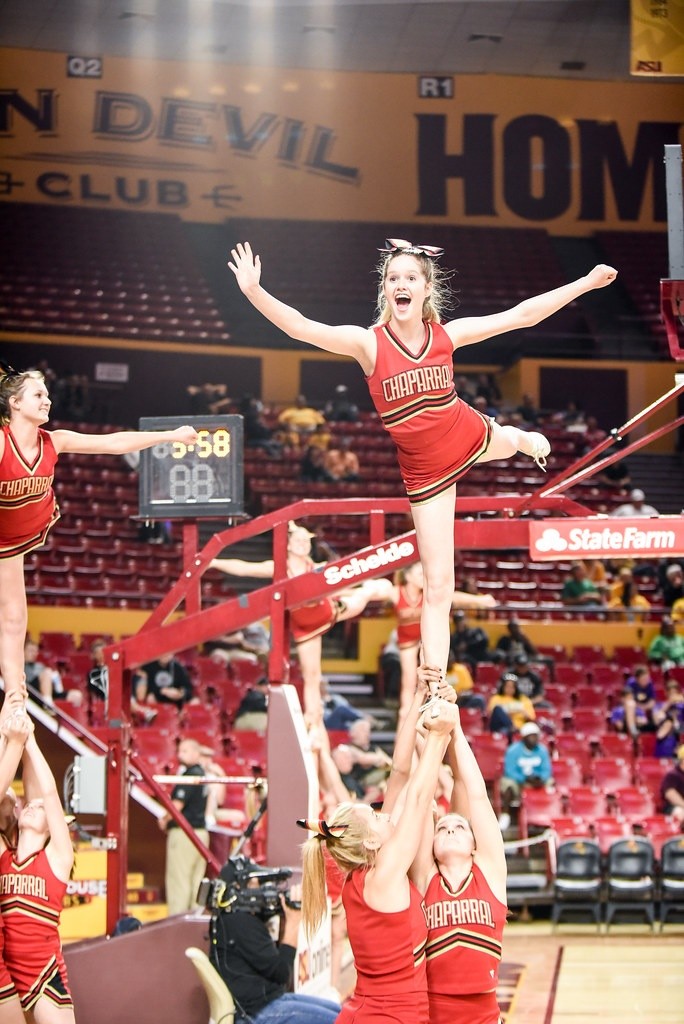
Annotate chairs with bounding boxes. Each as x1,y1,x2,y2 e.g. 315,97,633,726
21,409,678,625
23,631,684,938
0,198,684,363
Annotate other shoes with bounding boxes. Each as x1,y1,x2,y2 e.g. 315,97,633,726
4,709,34,733
418,693,458,719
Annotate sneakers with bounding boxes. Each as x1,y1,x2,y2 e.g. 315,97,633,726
501,425,551,473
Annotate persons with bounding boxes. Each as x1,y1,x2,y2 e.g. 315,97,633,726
227,238,619,716
199,372,684,1024
0,366,268,1024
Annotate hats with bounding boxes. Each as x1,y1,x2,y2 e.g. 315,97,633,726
520,722,539,737
219,856,256,882
